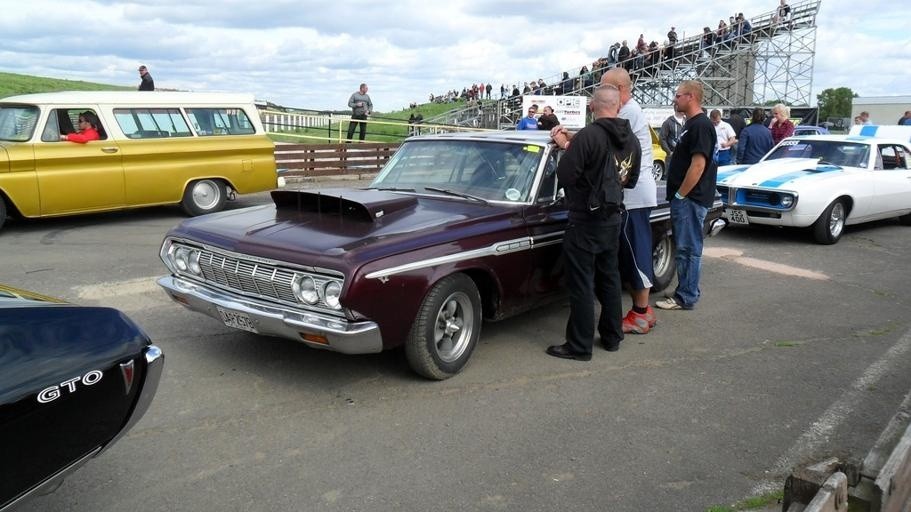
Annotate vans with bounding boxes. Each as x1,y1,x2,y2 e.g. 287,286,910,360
0,90,286,231
2,286,63,305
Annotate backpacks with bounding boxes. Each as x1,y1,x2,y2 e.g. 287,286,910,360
583,135,625,220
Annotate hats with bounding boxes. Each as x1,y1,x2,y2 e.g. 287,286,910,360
80,111,98,129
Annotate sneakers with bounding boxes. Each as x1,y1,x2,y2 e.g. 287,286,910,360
622,304,657,336
655,296,691,312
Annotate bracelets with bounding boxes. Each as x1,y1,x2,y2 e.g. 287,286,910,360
675,192,685,200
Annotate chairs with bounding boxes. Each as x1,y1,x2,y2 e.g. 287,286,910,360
470,161,505,189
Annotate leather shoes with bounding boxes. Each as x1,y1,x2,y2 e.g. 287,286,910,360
601,339,620,353
546,340,593,363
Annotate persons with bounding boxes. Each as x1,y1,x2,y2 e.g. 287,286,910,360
860,111,873,125
773,1,791,24
138,65,154,91
60,110,102,144
547,83,643,361
702,12,752,52
654,79,720,312
345,82,373,144
549,65,658,335
408,79,560,131
559,27,679,92
897,111,911,125
855,116,863,125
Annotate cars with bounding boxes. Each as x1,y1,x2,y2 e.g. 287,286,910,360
526,119,670,185
2,281,166,511
719,125,911,246
155,126,730,381
788,122,831,138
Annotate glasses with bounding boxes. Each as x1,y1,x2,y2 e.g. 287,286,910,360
675,94,689,100
